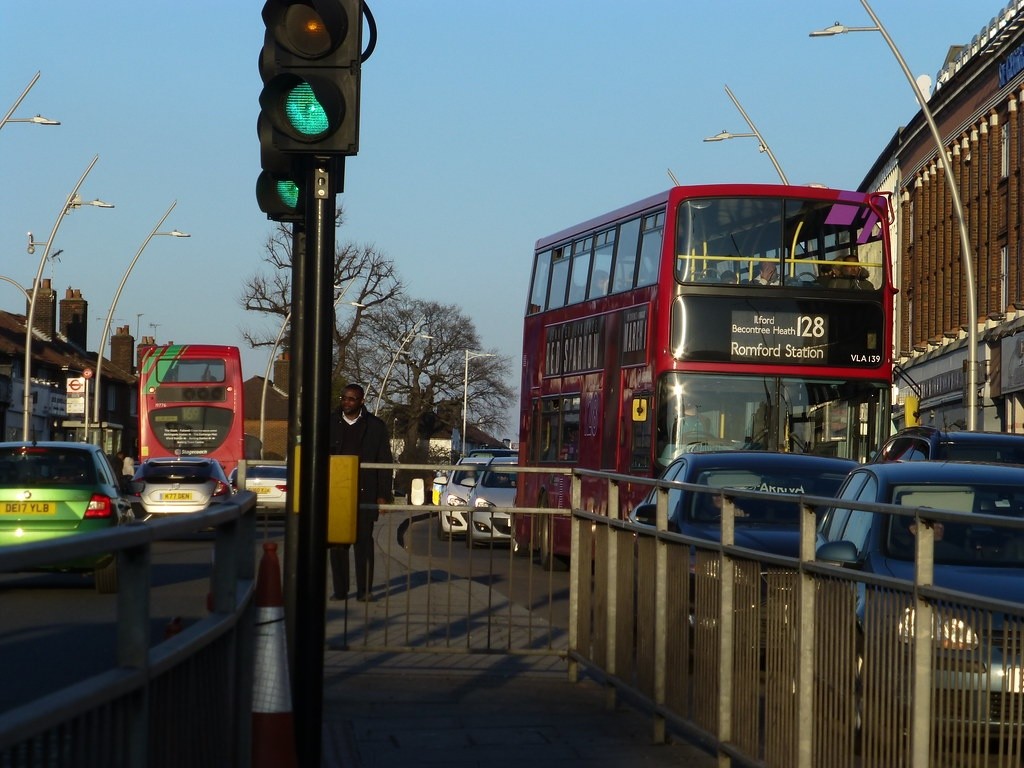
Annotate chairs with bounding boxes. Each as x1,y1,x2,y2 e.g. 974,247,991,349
687,268,818,288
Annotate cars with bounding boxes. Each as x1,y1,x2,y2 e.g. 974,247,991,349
621,448,864,674
787,462,1024,768
431,447,519,549
226,463,289,522
125,456,234,540
0,440,132,595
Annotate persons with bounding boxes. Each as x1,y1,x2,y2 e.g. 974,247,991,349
821,255,874,292
110,447,136,480
328,384,394,602
496,473,515,487
748,261,779,286
463,471,479,483
589,269,609,300
903,506,959,563
694,476,749,518
720,270,737,284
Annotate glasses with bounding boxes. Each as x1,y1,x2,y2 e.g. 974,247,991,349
341,396,361,402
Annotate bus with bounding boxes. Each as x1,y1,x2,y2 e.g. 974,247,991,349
512,182,900,572
136,343,245,486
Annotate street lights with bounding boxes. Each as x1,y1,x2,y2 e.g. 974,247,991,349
703,83,788,185
258,277,366,459
461,349,497,456
94,199,191,422
809,0,979,431
0,154,115,439
374,314,434,416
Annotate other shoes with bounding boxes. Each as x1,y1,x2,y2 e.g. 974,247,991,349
330,593,347,600
355,591,372,600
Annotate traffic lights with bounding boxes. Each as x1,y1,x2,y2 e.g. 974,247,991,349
256,109,336,223
261,0,361,152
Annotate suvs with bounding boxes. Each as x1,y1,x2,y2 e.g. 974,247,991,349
867,425,1023,470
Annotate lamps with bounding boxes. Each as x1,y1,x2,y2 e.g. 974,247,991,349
900,352,912,358
988,314,1004,321
927,340,942,347
961,326,968,332
944,332,958,340
913,346,927,353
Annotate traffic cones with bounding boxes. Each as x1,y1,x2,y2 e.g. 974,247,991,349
247,542,300,768
